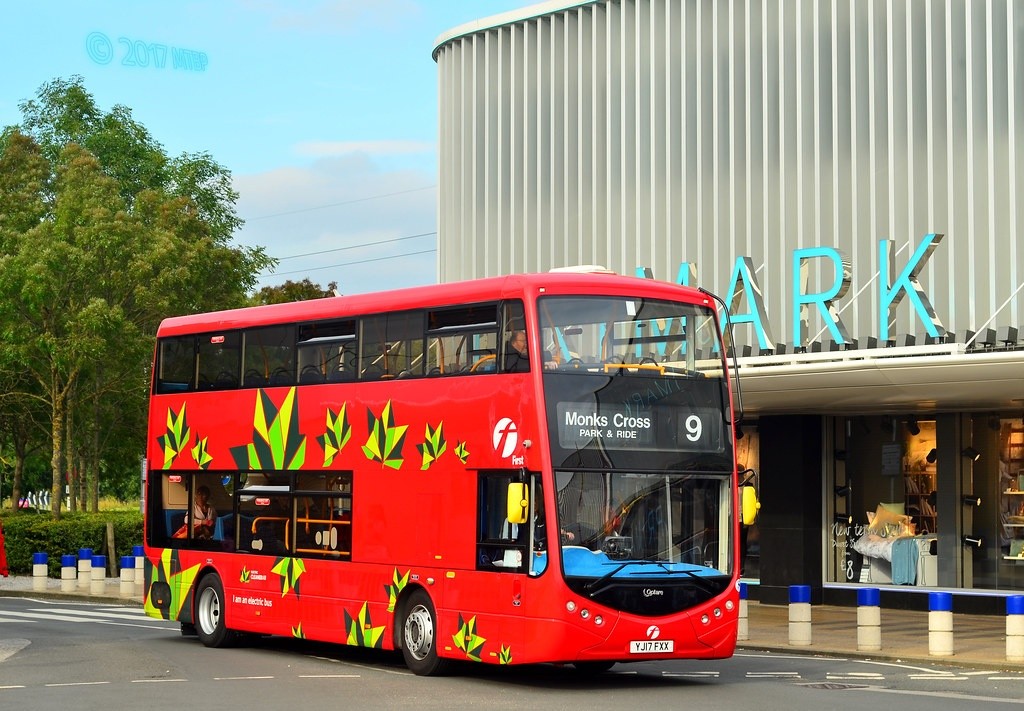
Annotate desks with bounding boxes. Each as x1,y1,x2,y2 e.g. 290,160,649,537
853,533,938,586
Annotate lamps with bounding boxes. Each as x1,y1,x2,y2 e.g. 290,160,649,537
830,415,939,556
966,533,982,551
989,411,1003,432
735,422,748,441
961,444,982,464
965,494,981,507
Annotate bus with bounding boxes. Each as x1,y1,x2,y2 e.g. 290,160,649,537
144,263,762,674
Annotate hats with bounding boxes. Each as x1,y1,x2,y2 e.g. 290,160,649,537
737,464,744,474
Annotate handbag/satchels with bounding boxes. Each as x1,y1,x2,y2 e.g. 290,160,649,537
171,524,204,539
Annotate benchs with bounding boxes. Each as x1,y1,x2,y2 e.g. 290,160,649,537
166,510,187,536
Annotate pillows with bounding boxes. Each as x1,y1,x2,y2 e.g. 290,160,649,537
866,502,916,537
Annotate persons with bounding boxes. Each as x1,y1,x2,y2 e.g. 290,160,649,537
304,494,332,520
184,485,218,540
503,330,559,374
500,483,575,568
738,463,753,576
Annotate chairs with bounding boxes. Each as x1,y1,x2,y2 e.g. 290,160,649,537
213,514,250,541
156,357,662,395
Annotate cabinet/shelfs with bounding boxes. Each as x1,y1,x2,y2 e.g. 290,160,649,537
906,471,937,532
998,425,1024,560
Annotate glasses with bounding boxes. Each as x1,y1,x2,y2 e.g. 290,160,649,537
517,340,527,344
